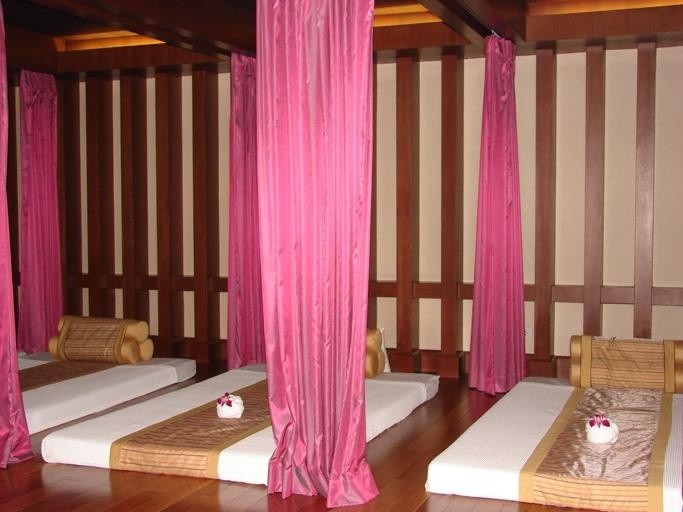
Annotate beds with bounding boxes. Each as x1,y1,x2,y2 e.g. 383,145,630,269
423,333,683,506
14,314,195,439
41,324,441,489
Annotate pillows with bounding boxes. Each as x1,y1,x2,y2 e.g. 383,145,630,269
364,328,387,380
56,313,154,364
566,332,683,395
